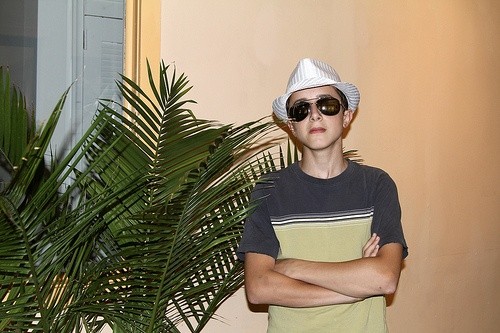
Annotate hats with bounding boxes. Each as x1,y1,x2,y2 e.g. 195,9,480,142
272,57,361,125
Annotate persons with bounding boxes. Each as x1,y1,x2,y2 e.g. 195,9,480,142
236,58,408,332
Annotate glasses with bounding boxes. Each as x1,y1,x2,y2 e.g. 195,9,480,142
286,97,348,122
